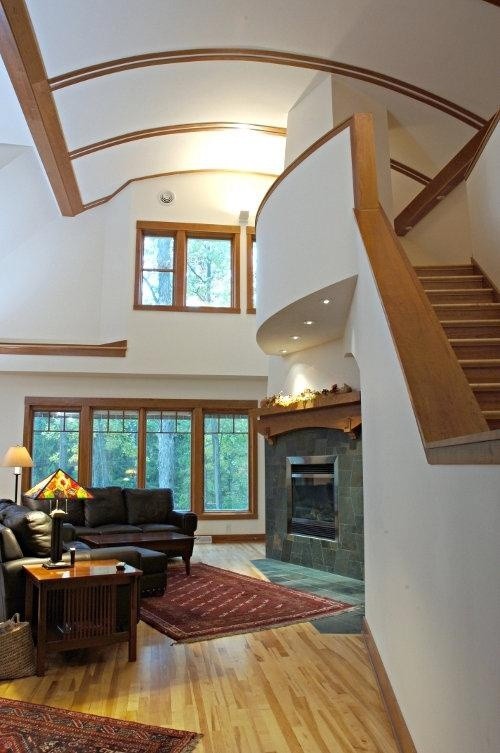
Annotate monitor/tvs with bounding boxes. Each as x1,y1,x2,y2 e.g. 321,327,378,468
291,474,335,524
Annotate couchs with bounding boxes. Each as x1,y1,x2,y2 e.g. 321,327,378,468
20,486,198,576
0,496,167,647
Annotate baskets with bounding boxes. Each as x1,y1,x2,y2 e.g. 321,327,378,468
0,613,37,681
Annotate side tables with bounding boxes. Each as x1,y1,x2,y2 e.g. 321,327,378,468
21,558,143,678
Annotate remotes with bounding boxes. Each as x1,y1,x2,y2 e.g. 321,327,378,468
116,560,127,571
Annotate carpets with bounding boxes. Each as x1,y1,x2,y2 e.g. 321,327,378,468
136,560,360,644
0,697,204,753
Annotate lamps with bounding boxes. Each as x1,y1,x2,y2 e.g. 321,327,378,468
0,444,35,506
23,468,94,570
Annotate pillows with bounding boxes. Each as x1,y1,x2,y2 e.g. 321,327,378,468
21,488,85,527
123,487,175,525
0,498,69,557
82,486,123,528
